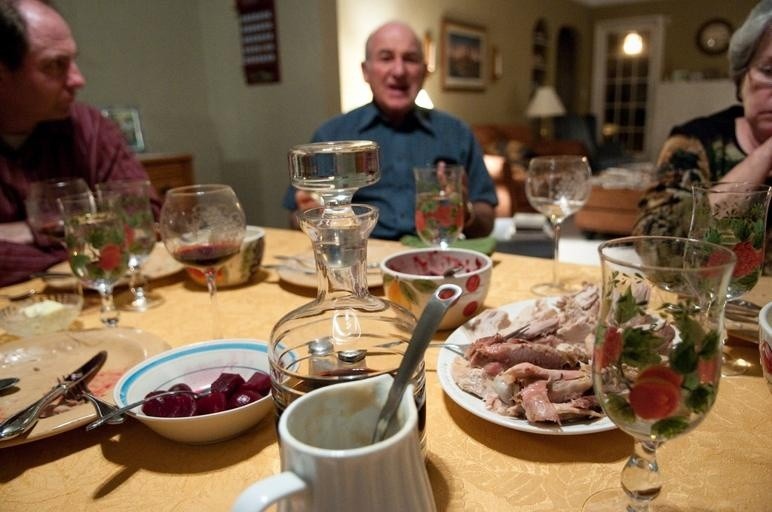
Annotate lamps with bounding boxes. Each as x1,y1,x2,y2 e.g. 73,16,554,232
526,86,567,141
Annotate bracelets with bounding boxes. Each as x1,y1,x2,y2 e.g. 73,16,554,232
464,202,476,232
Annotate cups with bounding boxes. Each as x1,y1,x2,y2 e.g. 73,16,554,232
225,380,440,509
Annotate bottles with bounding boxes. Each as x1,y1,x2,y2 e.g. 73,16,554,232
269,140,431,457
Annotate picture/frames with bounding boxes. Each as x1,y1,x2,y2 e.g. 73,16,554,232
101,106,146,153
440,17,494,92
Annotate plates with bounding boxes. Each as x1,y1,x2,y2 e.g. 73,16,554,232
276,250,393,286
1,330,173,449
439,299,618,438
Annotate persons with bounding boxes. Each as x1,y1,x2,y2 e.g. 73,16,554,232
282,21,499,242
0,0,163,289
631,0,772,285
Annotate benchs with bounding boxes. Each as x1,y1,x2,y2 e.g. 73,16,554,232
573,162,657,246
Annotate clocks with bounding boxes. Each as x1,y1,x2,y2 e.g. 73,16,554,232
696,17,735,55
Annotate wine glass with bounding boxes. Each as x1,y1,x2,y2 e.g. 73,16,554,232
413,161,467,250
580,235,737,512
158,185,249,327
526,155,592,296
690,179,771,381
57,178,166,336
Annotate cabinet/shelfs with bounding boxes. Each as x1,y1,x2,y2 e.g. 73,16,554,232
133,151,204,242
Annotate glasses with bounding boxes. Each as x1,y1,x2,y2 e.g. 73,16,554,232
746,62,772,87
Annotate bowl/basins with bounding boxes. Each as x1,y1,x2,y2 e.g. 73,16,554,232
4,295,85,332
121,339,301,448
379,251,492,330
183,227,266,288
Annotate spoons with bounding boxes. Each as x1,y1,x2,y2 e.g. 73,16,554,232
370,281,465,442
76,386,126,428
0,347,114,442
88,384,219,430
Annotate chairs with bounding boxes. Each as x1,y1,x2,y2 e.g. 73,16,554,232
470,123,593,213
